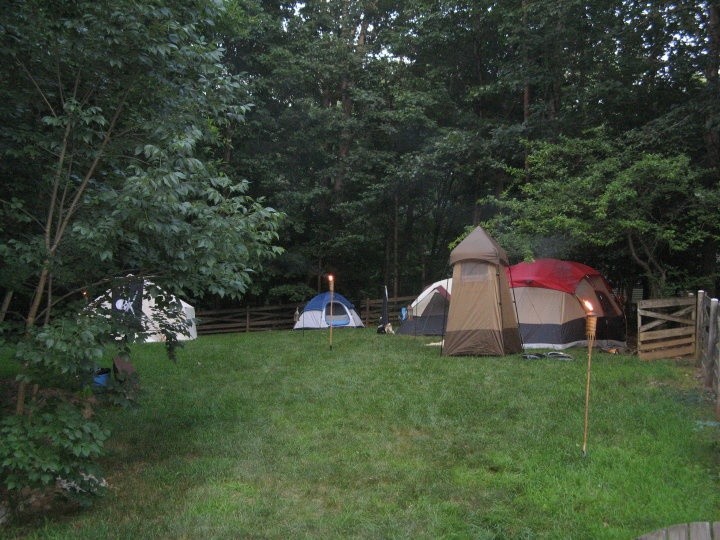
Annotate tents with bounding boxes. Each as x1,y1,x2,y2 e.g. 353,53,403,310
400,278,453,336
440,225,523,357
292,291,365,330
78,274,198,343
508,257,627,351
